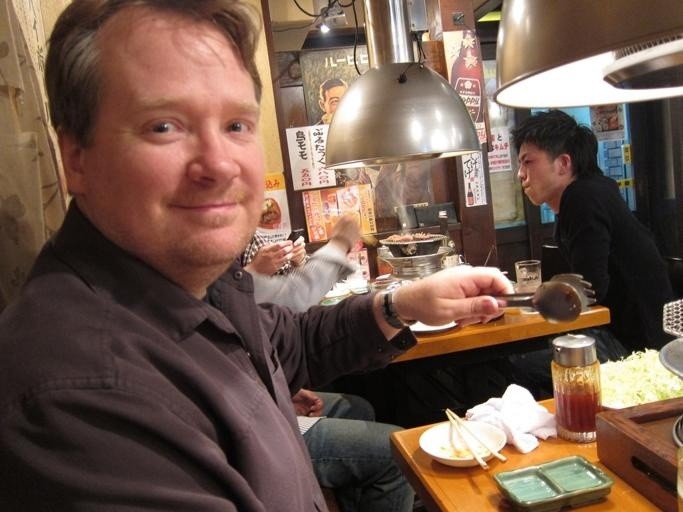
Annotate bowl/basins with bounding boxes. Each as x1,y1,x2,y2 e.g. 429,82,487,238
378,233,447,256
418,420,506,468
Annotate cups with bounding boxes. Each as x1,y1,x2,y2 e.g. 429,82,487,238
676,447,683,512
515,260,543,315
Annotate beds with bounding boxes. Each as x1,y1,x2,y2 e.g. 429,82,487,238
543,245,683,296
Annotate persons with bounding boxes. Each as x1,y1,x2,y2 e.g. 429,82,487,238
312,78,348,125
1,0,516,512
285,60,303,86
508,108,677,403
232,208,415,512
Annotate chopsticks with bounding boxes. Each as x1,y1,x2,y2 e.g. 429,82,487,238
445,407,507,470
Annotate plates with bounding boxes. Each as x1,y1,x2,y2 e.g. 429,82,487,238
405,320,460,333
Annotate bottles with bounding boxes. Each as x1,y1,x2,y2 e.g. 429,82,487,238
438,211,458,270
551,334,603,444
449,28,484,123
467,182,474,205
376,245,394,276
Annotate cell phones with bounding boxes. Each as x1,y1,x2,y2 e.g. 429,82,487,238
287,228,305,241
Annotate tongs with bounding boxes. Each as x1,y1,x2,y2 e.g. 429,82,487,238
478,273,597,325
